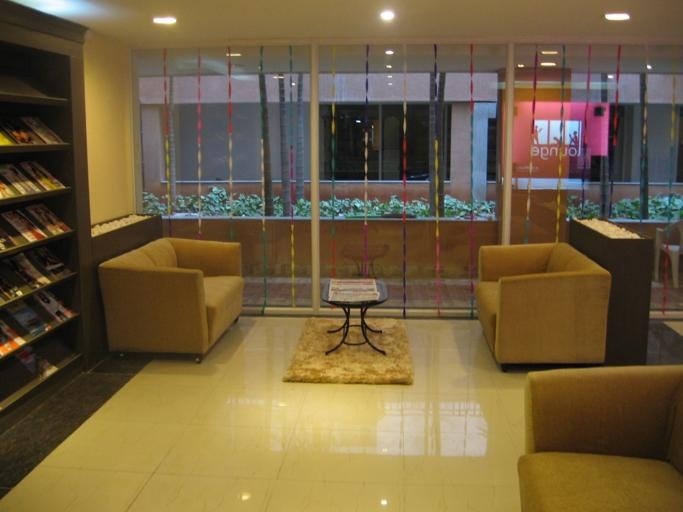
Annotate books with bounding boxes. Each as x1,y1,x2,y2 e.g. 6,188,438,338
0,116,80,403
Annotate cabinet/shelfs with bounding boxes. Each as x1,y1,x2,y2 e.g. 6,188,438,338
0,0,98,434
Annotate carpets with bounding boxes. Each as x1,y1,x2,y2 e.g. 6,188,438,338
283,318,414,385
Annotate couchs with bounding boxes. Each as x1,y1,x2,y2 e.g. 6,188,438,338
98,237,244,362
517,364,683,512
474,242,612,372
